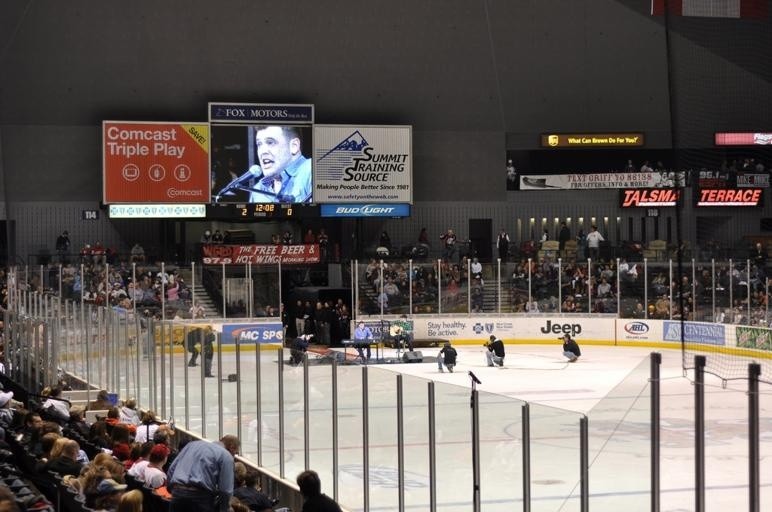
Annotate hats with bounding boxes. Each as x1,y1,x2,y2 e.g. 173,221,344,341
152,425,175,457
69,405,87,416
94,478,127,497
0,390,14,408
39,386,51,400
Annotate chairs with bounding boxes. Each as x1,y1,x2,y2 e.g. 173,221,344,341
0,384,184,512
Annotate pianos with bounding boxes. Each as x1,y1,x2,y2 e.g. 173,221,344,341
341,339,380,344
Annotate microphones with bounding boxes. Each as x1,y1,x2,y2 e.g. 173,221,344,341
218,165,262,196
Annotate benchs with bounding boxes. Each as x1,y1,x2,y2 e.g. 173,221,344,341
383,338,450,348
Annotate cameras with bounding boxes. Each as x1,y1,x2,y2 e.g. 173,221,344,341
305,334,313,339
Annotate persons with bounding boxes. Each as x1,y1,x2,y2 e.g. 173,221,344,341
0,225,348,348
0,325,347,511
435,342,457,372
562,335,581,362
483,335,505,367
352,320,374,363
248,123,313,202
393,315,413,353
348,221,772,326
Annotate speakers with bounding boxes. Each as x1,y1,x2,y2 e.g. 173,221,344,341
403,351,423,363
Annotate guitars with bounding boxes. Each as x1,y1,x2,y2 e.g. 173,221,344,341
390,325,416,337
